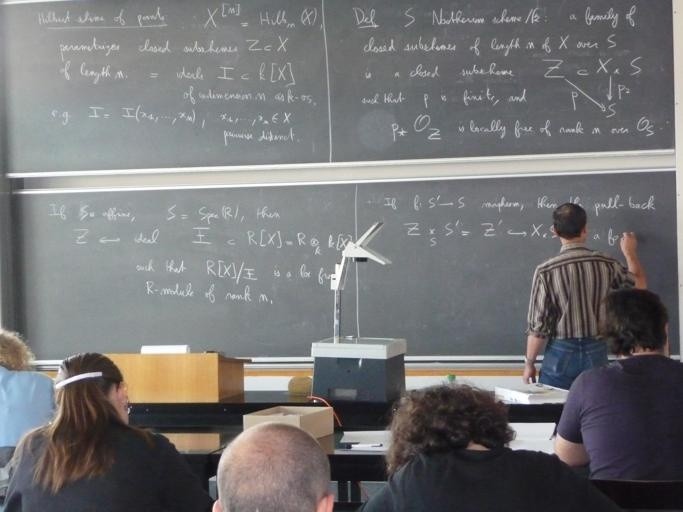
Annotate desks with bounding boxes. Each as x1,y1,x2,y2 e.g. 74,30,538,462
125,431,562,504
127,389,565,432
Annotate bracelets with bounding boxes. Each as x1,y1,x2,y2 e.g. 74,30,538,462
524,356,535,364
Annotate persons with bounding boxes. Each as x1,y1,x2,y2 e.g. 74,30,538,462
2,354,215,512
558,289,683,483
1,330,58,452
212,424,335,511
524,203,649,389
363,383,618,511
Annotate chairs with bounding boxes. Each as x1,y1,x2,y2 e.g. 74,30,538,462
588,478,682,512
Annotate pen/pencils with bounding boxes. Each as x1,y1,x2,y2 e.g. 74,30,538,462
346,443,383,449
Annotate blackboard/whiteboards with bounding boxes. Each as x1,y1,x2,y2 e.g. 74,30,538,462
10,170,679,360
0,0,675,173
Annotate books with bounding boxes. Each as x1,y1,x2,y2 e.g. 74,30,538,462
498,383,570,406
